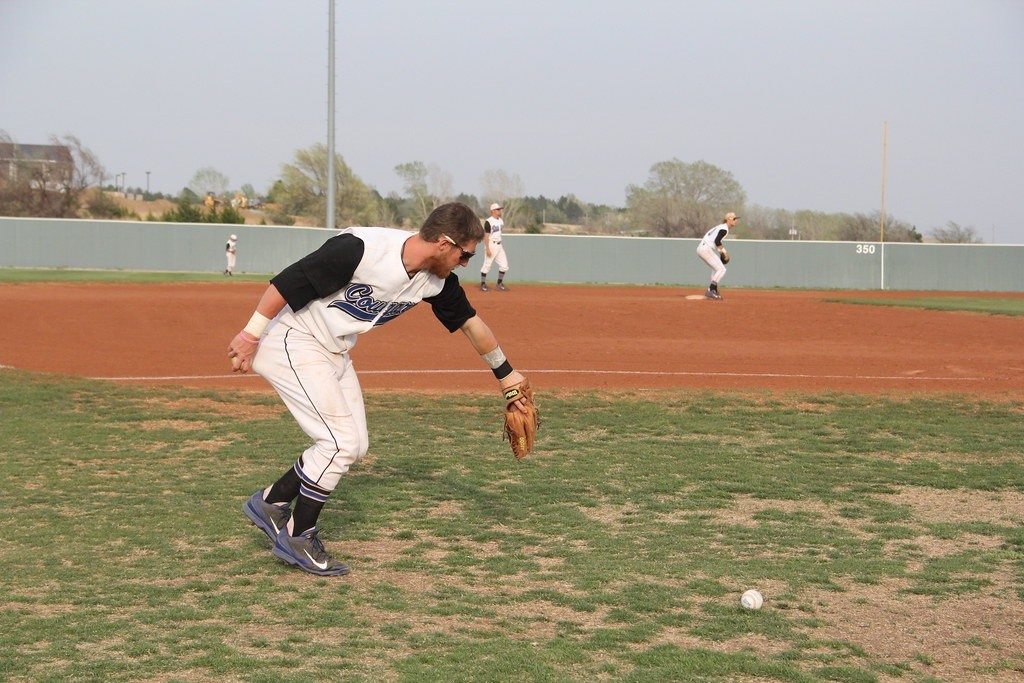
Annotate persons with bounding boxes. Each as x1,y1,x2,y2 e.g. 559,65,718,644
697,212,741,300
227,202,537,575
224,235,237,276
480,203,509,291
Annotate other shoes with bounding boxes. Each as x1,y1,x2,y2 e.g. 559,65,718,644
481,287,488,291
705,290,722,300
226,269,232,277
496,284,506,290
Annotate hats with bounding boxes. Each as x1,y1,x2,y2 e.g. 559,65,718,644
724,212,741,219
490,203,504,210
230,235,238,242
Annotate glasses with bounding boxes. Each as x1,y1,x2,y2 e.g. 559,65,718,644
442,233,477,261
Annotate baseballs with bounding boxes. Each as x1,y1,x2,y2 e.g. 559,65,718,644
740,590,763,611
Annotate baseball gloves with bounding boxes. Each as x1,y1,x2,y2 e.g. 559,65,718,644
500,381,540,459
720,248,729,265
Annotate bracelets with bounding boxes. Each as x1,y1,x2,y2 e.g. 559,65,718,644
480,344,514,380
722,248,726,253
244,311,269,339
240,331,259,345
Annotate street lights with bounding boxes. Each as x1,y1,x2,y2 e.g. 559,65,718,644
121,172,126,192
145,171,151,192
115,174,120,192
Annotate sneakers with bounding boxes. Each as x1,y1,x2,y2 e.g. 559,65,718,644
242,487,292,543
272,524,350,576
